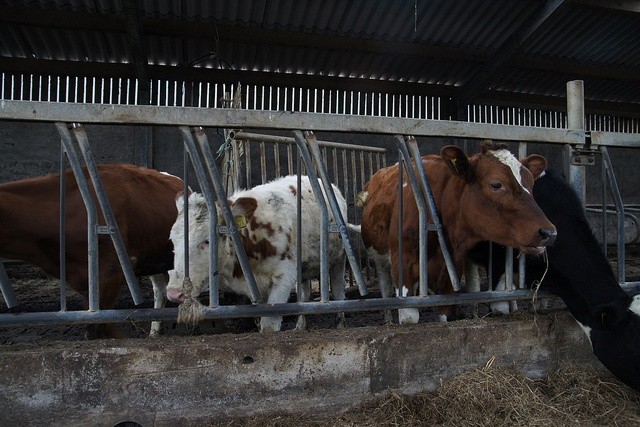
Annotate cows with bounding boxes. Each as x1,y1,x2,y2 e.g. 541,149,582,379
463,167,640,395
354,139,558,327
0,162,194,341
165,174,362,334
